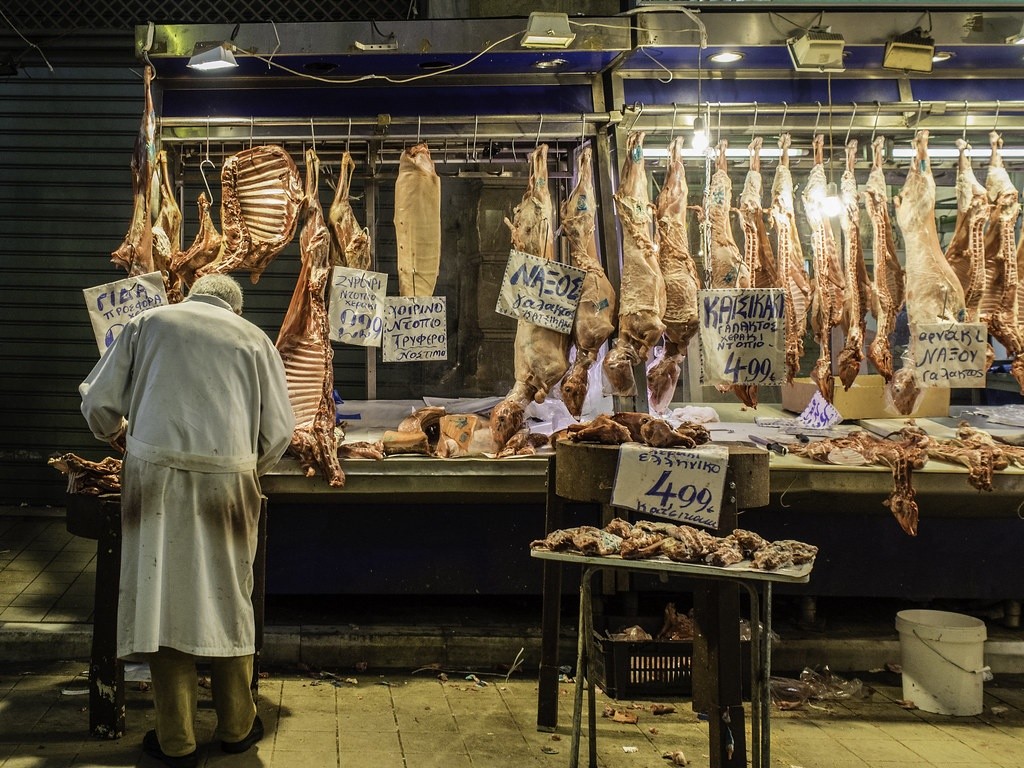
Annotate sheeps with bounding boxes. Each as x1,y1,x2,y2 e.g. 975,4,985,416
490,129,1024,449
793,424,1009,537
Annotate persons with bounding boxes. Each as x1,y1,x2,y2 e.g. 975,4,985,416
78,273,297,768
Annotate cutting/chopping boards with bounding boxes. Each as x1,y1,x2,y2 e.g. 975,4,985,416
64,487,124,538
555,440,770,509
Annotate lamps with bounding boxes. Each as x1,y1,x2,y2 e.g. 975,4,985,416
789,33,848,73
881,143,1024,159
521,10,577,48
642,143,810,157
883,36,937,75
186,41,239,70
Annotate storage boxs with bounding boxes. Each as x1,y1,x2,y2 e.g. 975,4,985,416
590,628,751,700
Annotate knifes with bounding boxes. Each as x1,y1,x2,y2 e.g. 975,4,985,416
749,435,786,454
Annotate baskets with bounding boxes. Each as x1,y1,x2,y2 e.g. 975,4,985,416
592,630,762,701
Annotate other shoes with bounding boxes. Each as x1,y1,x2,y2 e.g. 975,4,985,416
143,729,198,768
221,716,264,753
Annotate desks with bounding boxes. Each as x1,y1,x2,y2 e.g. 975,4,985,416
87,456,561,739
769,468,1024,657
530,548,815,768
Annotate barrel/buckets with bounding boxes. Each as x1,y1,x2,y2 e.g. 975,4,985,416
895,609,994,716
